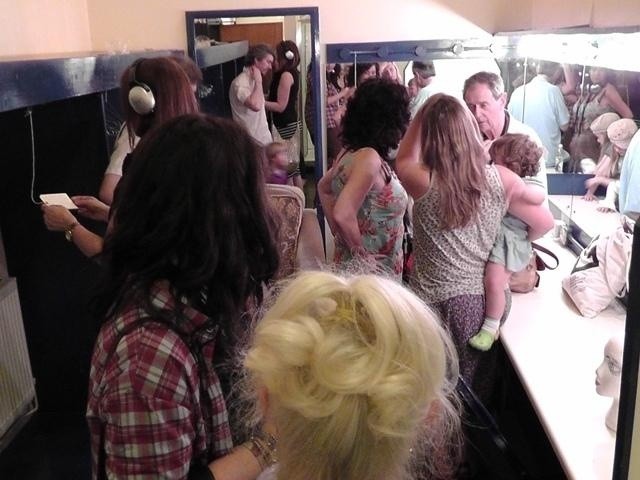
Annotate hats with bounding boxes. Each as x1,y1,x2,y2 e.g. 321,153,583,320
607,118,638,150
590,112,621,134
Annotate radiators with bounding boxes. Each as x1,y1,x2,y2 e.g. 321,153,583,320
1,276,35,444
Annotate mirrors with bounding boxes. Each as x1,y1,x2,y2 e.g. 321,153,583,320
498,58,640,316
184,3,330,263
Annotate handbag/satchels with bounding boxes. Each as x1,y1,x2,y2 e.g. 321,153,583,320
272,122,300,173
508,242,538,292
563,225,632,319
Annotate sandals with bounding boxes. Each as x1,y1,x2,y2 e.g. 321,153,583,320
469,317,500,352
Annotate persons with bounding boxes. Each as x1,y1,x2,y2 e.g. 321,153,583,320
228,43,276,148
507,59,640,223
595,336,625,431
265,40,303,190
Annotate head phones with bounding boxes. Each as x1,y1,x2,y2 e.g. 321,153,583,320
586,83,601,95
126,57,157,116
280,40,295,61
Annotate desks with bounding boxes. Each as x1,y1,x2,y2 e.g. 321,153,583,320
495,224,629,480
